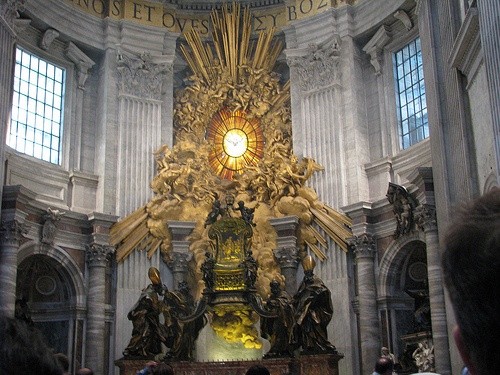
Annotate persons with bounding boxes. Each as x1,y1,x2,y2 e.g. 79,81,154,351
150,58,325,227
243,249,258,290
293,255,337,355
137,361,174,375
245,365,270,375
411,341,436,372
162,279,199,361
442,189,500,375
200,251,214,291
75,367,93,375
381,347,396,364
0,316,64,375
123,267,162,360
260,275,295,357
371,356,394,375
